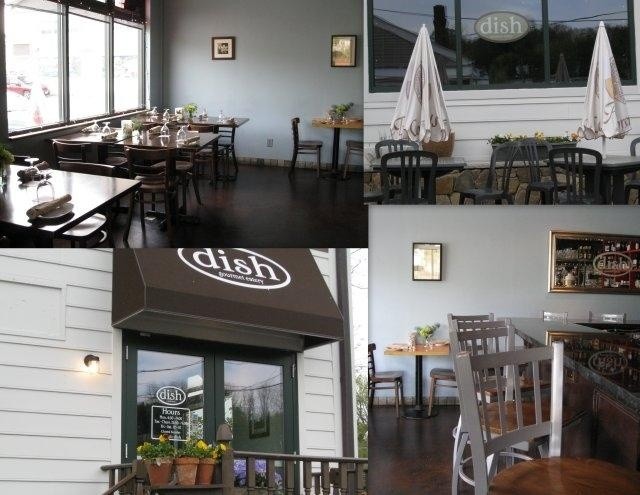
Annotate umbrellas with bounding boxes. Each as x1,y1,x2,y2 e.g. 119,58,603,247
554,52,569,84
576,18,631,159
434,49,448,87
389,23,450,197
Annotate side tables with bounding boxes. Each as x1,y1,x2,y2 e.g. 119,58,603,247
313,119,363,181
384,343,452,422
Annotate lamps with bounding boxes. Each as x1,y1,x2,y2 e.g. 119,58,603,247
83,353,102,373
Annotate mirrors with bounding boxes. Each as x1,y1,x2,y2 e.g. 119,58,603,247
412,242,444,283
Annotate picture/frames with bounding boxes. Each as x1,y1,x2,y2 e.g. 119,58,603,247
330,33,358,69
210,36,235,60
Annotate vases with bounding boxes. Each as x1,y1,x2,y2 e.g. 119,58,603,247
173,458,200,487
144,456,173,487
195,458,218,487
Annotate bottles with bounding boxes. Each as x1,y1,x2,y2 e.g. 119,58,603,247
556,239,640,290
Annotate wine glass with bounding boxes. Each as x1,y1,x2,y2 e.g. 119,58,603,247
36,169,55,201
23,157,41,188
150,106,225,141
92,120,99,131
101,122,111,135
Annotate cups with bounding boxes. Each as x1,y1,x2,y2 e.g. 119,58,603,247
121,120,133,137
563,130,570,138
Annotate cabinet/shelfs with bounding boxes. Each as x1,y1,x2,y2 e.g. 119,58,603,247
547,230,640,294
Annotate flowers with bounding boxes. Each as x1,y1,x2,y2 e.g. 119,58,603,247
138,435,177,460
173,439,209,460
233,458,283,490
211,445,227,461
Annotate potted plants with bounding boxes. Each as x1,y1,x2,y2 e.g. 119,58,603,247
328,100,354,123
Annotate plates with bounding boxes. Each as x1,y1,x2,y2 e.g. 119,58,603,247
384,345,401,351
27,201,74,220
432,340,449,346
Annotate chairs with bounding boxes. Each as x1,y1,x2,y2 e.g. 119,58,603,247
0,100,249,248
342,137,363,180
427,345,474,421
367,342,405,420
288,117,324,178
366,129,640,206
441,312,640,495
